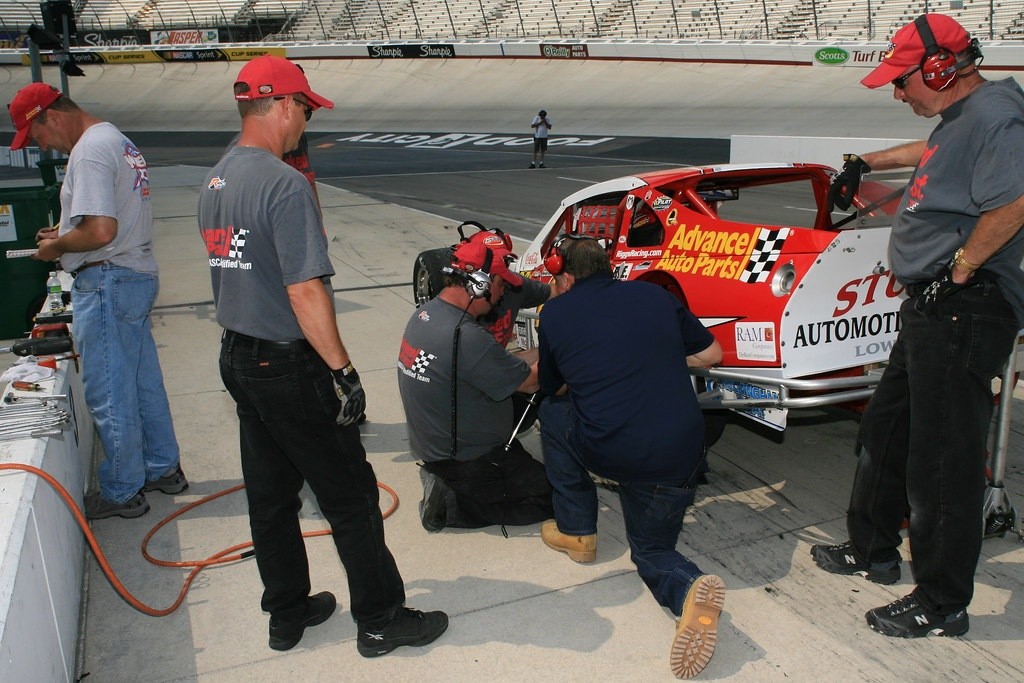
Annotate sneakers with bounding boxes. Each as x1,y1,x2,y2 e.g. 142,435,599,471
865,594,969,639
141,463,188,494
83,491,150,519
810,540,901,585
540,519,598,563
670,575,726,680
269,591,337,651
357,605,448,658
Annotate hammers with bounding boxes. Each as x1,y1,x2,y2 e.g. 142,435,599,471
4,392,67,405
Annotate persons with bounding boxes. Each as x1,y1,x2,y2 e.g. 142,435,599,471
10,82,188,519
199,55,449,659
539,237,726,681
397,242,552,532
810,15,1023,636
529,110,552,168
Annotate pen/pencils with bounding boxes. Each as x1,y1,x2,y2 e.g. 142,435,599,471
36,223,60,246
53,308,66,315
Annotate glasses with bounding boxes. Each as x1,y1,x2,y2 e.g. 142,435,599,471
892,66,923,89
274,96,313,122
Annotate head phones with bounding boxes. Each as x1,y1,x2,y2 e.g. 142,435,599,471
915,14,959,92
544,234,596,276
442,266,492,299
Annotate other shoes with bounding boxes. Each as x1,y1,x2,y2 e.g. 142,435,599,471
539,164,546,168
529,164,535,168
418,473,447,534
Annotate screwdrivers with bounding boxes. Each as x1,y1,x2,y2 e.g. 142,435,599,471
12,381,47,391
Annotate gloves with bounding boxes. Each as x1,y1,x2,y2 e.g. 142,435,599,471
917,269,964,312
330,362,366,427
828,154,872,212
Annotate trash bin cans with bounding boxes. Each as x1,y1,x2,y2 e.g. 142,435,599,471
1,181,63,342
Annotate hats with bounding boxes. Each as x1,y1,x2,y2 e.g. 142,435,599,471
451,243,523,287
235,54,334,110
861,14,973,89
10,82,63,151
469,232,518,259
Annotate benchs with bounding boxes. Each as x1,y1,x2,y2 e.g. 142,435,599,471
0,0,1024,41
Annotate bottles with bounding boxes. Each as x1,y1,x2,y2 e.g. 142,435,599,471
46,272,63,311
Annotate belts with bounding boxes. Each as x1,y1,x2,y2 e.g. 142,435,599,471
225,331,309,349
71,260,104,279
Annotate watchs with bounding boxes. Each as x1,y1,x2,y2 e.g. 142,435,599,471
954,247,981,271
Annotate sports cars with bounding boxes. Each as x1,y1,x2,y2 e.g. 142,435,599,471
412,162,910,434
